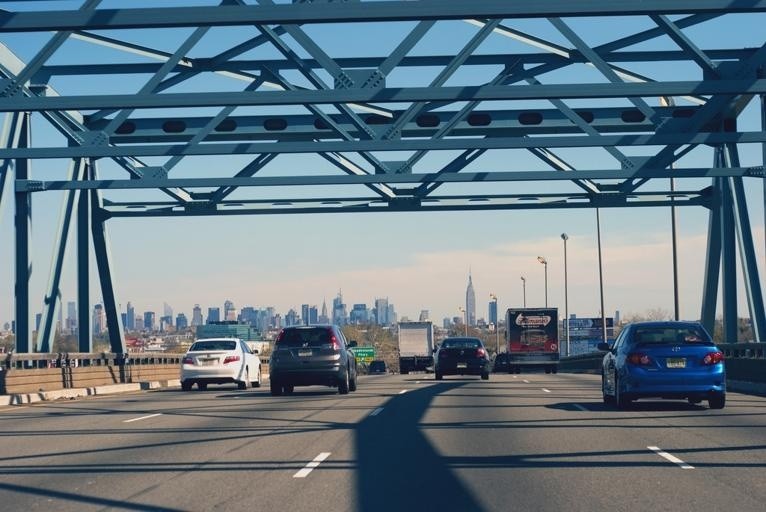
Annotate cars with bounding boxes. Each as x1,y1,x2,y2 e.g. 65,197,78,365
597,320,727,409
433,336,491,380
180,337,262,391
269,323,358,396
490,353,506,374
368,360,389,375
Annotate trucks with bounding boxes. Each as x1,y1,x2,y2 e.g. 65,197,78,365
505,307,561,374
397,322,434,374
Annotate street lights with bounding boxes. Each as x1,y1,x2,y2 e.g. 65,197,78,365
489,291,500,355
520,231,570,356
656,85,680,321
458,306,468,337
